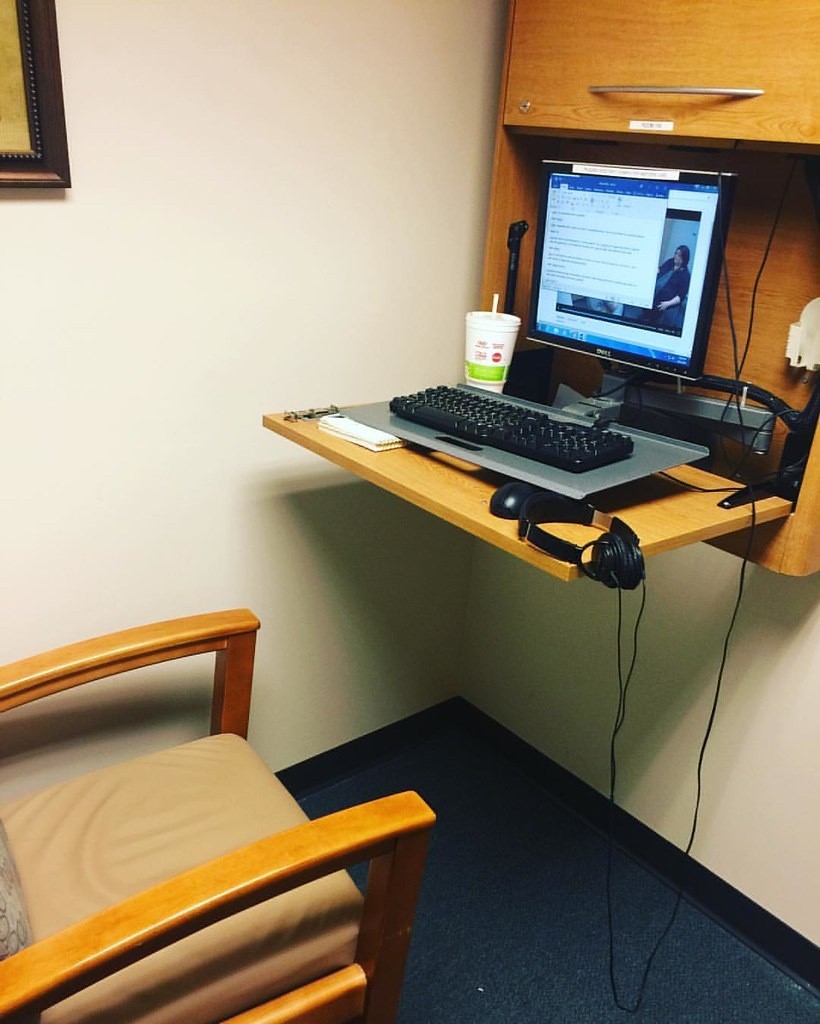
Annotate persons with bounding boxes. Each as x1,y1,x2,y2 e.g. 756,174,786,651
637,245,691,326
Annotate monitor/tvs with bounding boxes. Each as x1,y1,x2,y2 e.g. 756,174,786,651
525,160,741,381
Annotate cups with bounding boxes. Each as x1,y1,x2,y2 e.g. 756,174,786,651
465,312,521,394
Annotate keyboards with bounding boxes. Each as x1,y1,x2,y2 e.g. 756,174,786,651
388,384,634,473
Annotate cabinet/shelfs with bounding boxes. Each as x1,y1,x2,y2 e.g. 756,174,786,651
262,0,820,583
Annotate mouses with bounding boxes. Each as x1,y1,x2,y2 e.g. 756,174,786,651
490,481,540,520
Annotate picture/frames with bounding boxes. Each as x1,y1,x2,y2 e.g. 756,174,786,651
0,0,72,188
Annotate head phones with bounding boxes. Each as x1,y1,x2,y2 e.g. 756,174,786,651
518,492,646,591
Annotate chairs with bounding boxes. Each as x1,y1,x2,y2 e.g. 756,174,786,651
0,608,436,1024
